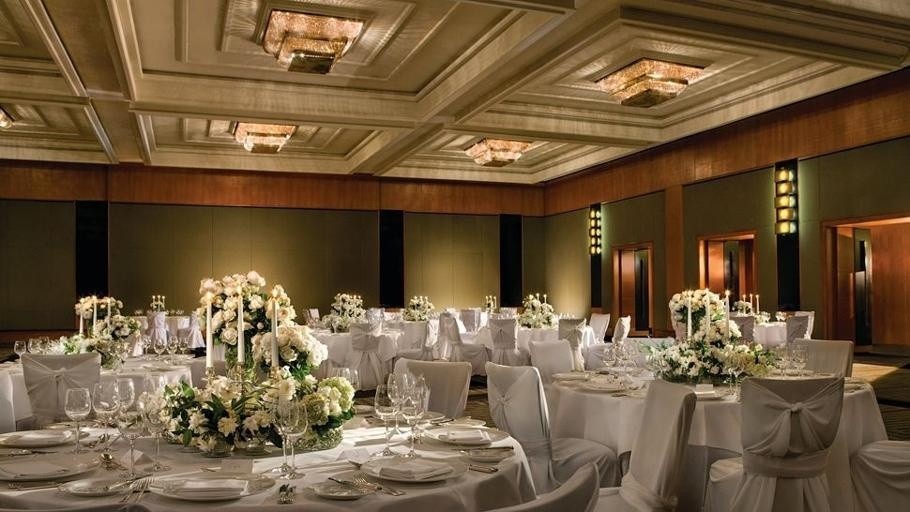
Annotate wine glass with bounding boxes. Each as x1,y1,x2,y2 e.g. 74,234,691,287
143,335,189,368
602,342,630,383
65,372,173,480
374,372,429,458
14,336,50,366
774,337,807,380
271,393,308,479
724,349,744,397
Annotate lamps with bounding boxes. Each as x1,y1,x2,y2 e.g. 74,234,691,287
228,120,298,154
252,0,377,75
589,209,601,256
775,169,797,236
574,49,715,108
458,136,537,168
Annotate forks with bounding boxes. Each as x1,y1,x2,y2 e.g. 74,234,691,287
352,475,383,492
118,478,154,505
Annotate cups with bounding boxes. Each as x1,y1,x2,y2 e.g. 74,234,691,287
331,367,359,393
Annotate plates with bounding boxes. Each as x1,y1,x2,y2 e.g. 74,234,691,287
551,372,627,393
425,410,509,446
691,391,726,400
351,404,374,415
359,457,469,484
312,479,377,500
0,429,90,447
0,453,276,501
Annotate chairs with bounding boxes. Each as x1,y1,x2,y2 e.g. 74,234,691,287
1,307,910,511
